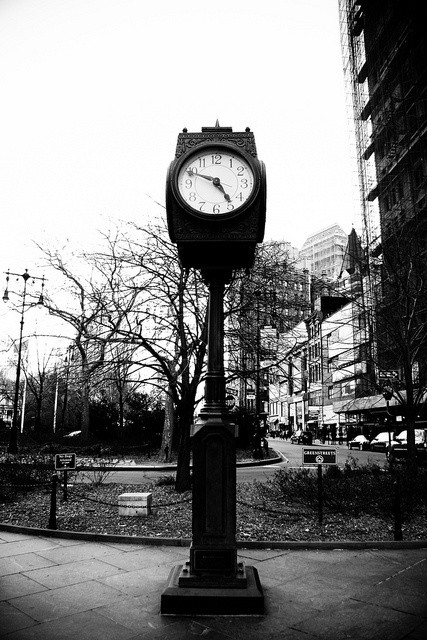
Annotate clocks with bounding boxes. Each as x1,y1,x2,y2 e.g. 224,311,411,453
162,121,268,282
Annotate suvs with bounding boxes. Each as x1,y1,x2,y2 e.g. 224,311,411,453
370,432,400,452
385,429,427,465
290,431,312,445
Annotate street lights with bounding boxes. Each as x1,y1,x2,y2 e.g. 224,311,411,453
3,269,48,454
62,345,77,423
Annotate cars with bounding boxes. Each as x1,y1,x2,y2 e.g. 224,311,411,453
348,435,376,450
